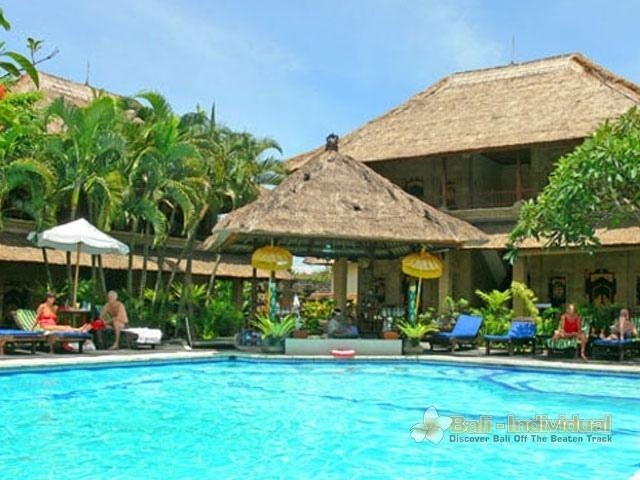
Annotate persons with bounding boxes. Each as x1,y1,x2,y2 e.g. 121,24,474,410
343,316,359,338
98,290,129,351
29,292,92,333
599,309,637,341
327,308,343,338
554,304,588,361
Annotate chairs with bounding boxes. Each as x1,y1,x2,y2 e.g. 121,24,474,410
1,305,162,354
428,314,639,363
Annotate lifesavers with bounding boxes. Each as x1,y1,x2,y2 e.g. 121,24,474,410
331,348,355,358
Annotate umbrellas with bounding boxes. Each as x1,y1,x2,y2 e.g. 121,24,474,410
39,217,131,308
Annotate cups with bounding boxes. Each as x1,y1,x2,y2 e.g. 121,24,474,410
74,302,80,310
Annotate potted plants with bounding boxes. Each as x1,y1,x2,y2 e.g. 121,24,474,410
247,312,298,352
390,315,440,354
